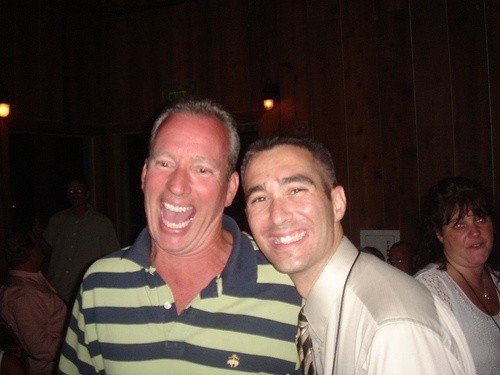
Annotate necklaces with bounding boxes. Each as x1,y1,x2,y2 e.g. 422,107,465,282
470,279,494,301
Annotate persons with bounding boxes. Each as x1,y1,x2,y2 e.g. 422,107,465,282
0,174,121,375
241,134,475,375
358,242,416,278
412,172,500,375
58,97,307,374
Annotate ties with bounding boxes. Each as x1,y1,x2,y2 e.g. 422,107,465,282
294,304,318,374
